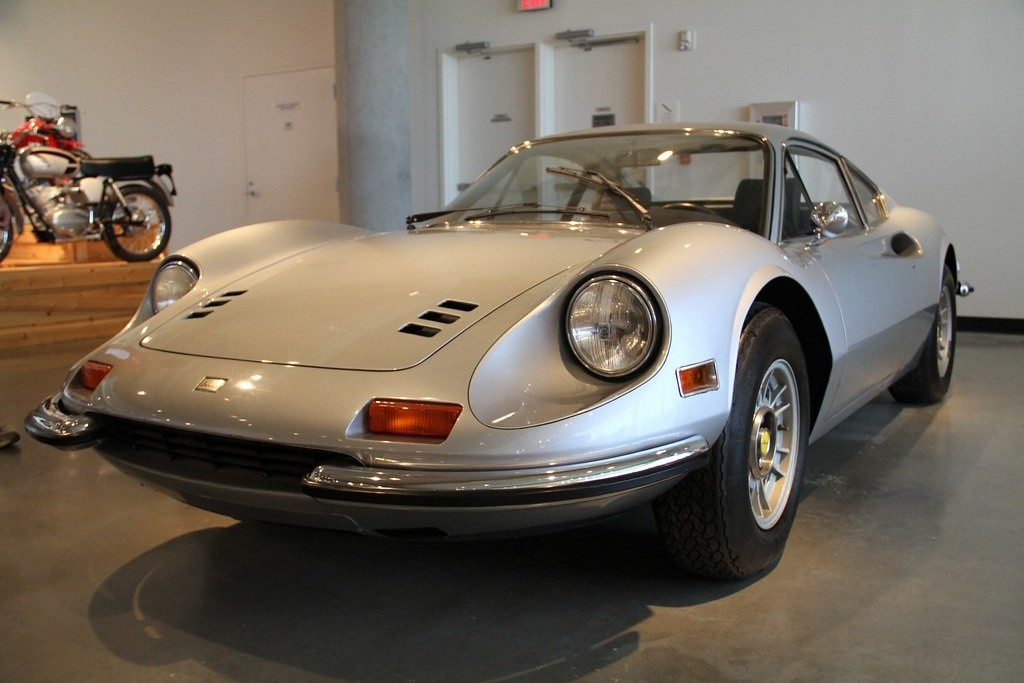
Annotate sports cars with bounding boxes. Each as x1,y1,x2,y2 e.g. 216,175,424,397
26,119,973,581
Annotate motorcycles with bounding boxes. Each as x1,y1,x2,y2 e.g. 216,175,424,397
0,99,177,263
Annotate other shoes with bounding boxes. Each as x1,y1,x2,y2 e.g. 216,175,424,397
0,431,21,448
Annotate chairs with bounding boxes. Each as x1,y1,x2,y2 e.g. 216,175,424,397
591,187,651,211
732,178,802,238
